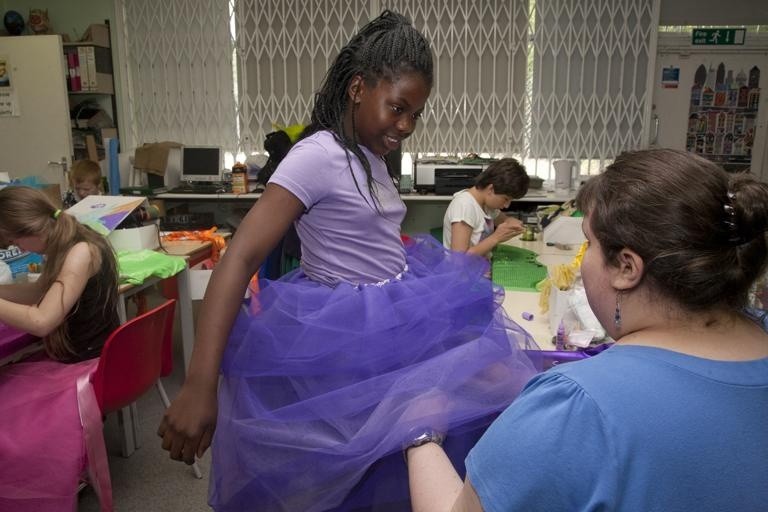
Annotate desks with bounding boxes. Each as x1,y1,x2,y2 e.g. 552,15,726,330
148,178,578,237
0,231,229,459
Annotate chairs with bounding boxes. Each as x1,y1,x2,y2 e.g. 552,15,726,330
90,300,205,480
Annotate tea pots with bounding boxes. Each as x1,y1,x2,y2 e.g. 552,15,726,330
553,159,578,196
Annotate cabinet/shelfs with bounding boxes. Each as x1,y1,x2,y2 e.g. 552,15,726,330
62,19,124,153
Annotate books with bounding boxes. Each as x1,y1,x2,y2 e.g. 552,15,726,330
64,46,98,92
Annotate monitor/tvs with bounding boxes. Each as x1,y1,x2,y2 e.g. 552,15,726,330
180,145,222,187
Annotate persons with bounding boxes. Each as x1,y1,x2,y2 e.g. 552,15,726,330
157,10,433,512
63,161,103,209
443,158,530,259
402,149,767,512
0,186,119,364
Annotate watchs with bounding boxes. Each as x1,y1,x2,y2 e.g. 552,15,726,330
402,426,445,464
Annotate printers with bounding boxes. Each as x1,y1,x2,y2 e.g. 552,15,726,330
414,158,498,196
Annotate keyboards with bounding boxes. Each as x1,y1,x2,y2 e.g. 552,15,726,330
166,187,221,194
